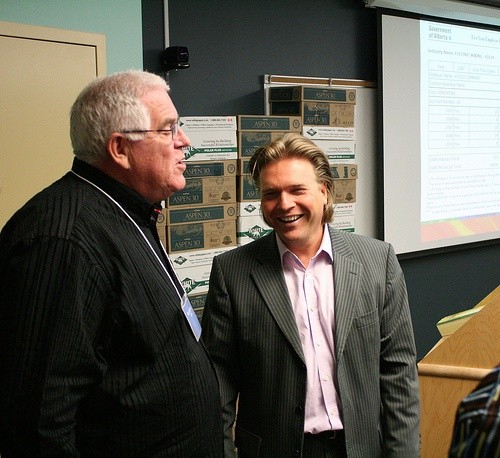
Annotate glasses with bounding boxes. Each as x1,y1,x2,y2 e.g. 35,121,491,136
122,119,184,141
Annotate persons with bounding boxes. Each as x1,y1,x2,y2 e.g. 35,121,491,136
0,69,225,458
447,363,500,458
200,131,420,458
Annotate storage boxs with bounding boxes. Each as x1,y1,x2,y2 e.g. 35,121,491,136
155,85,356,326
437,307,486,337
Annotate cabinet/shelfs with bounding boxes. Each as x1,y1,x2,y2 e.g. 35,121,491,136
416,286,500,458
